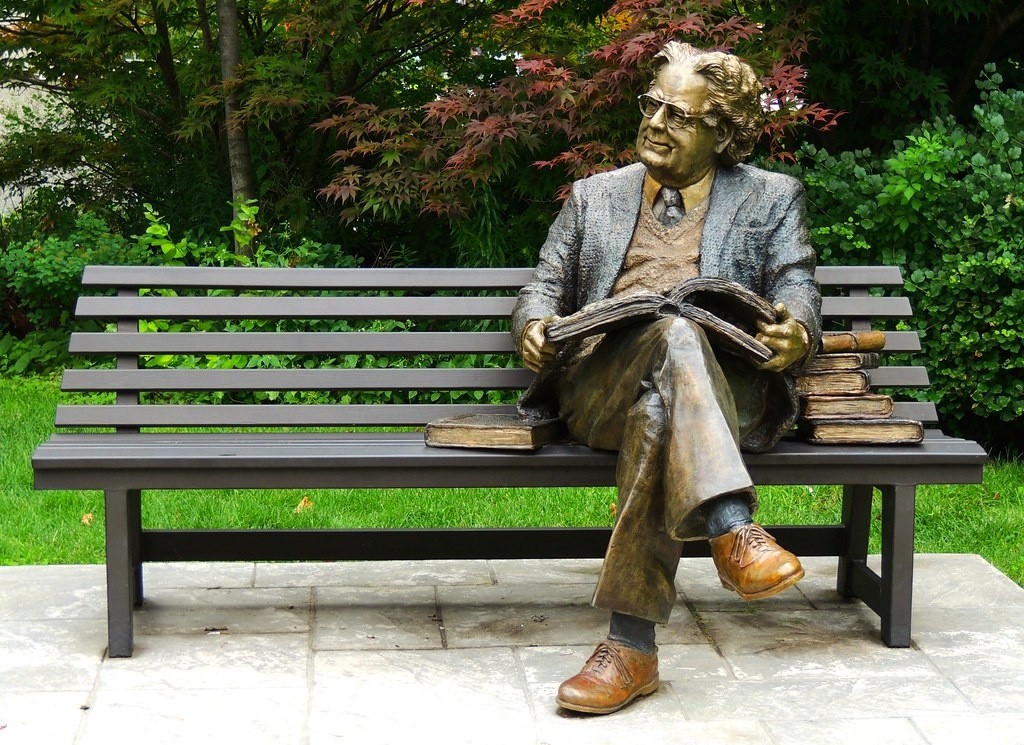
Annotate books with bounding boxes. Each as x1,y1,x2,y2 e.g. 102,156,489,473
792,331,924,443
546,276,778,360
424,411,562,451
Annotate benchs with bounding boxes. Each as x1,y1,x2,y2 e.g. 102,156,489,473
30,265,988,647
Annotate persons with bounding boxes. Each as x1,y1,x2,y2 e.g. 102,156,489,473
510,42,822,713
